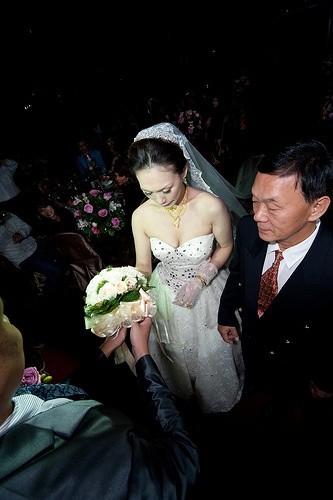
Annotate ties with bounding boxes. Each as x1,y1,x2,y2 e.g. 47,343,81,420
258,250,284,319
13,384,88,400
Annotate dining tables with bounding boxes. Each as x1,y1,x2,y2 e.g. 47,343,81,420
61,164,133,205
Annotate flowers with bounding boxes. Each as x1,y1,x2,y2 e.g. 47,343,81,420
82,264,157,356
72,189,126,240
178,110,203,135
21,366,53,386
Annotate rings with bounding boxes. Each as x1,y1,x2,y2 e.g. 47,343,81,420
188,304,192,309
182,299,186,304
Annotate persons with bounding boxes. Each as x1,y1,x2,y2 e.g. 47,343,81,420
0,158,86,283
218,140,333,500
0,318,201,500
77,135,146,207
131,122,245,415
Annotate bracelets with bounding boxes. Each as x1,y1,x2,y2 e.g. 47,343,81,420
194,275,206,286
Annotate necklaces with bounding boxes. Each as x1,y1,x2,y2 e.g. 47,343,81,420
165,188,189,229
